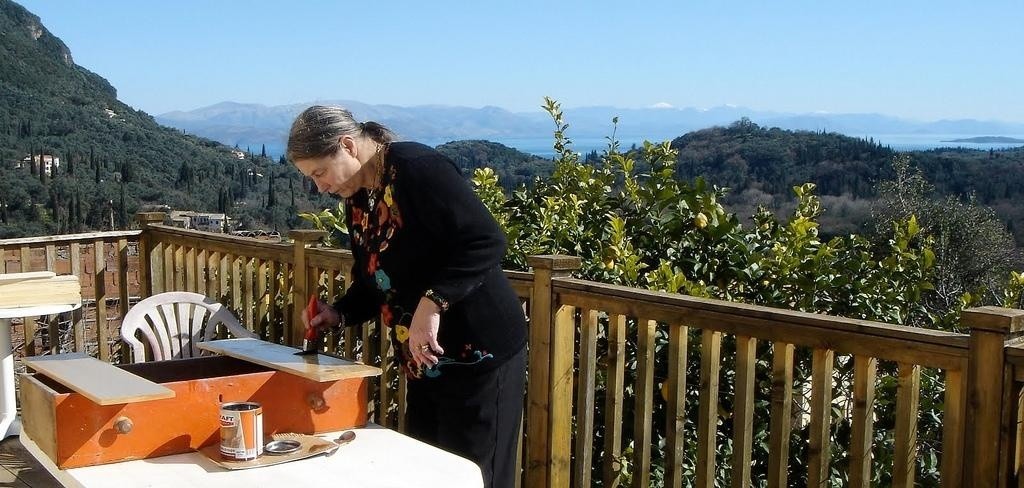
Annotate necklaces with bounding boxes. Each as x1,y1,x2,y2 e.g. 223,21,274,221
365,142,384,212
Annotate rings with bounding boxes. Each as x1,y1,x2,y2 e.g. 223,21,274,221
415,345,429,353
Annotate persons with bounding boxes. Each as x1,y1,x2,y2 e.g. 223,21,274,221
286,105,530,487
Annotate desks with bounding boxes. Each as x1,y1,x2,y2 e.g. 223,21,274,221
19,422,484,488
0,277,82,442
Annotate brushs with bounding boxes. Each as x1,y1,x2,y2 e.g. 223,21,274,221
292,295,318,355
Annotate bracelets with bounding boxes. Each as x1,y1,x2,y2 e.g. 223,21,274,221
331,313,343,332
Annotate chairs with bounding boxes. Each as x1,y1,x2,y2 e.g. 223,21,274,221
120,291,261,364
